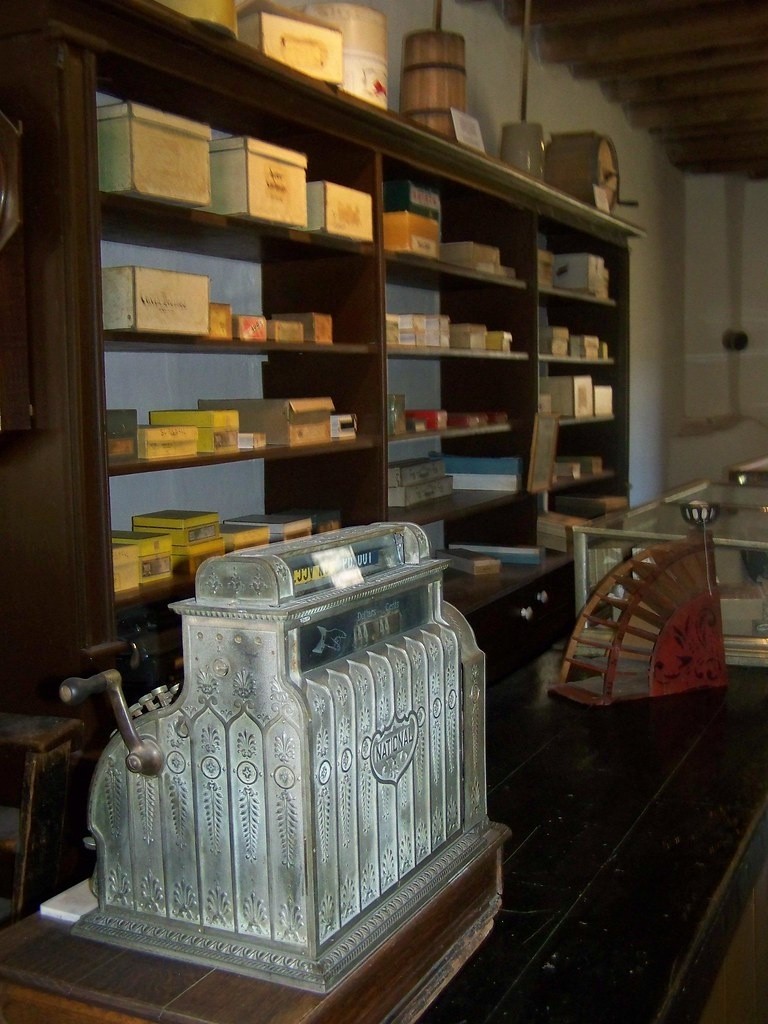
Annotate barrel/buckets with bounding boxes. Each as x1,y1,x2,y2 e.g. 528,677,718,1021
401,32,466,138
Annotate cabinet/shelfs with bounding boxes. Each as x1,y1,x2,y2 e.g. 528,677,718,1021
0,710,81,926
0,2,645,716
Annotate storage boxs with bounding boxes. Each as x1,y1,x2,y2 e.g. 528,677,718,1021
539,376,614,420
101,267,210,334
331,414,356,438
105,410,266,459
97,103,212,208
435,492,628,574
208,301,332,345
199,397,331,445
112,508,340,591
307,179,374,243
550,455,604,485
386,313,512,352
383,178,518,277
209,136,308,228
388,394,509,436
387,451,522,507
539,326,608,358
539,250,609,298
236,0,347,86
